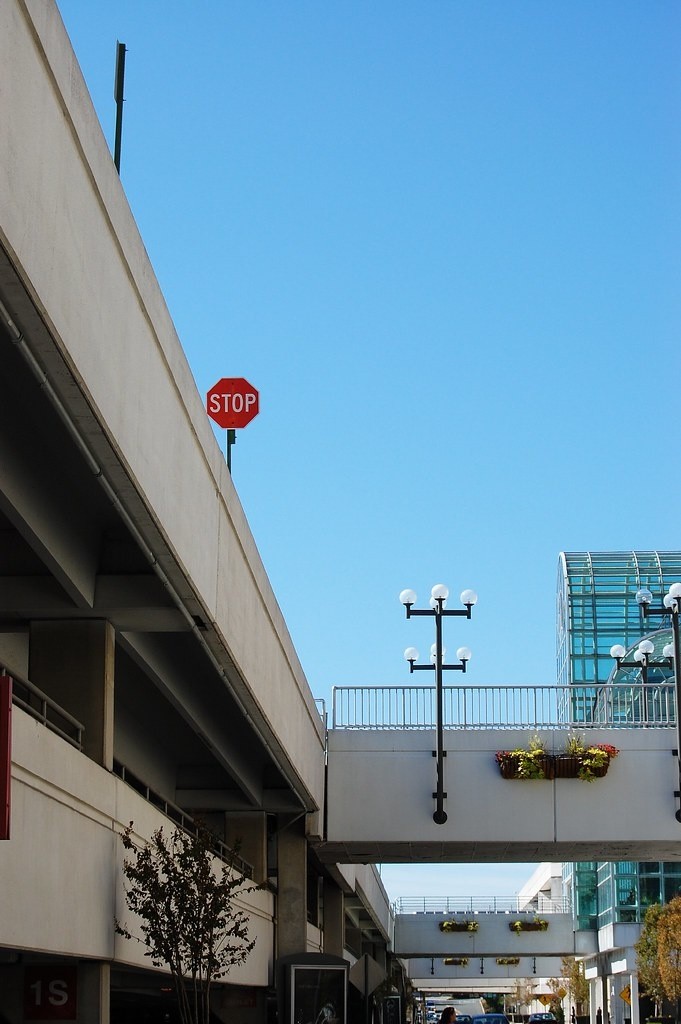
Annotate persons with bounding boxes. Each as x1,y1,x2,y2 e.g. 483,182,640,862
437,1007,456,1024
572,1006,575,1024
596,1007,602,1024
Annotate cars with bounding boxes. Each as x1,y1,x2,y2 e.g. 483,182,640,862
455,1015,471,1024
417,1002,443,1021
471,1014,509,1024
526,1013,559,1024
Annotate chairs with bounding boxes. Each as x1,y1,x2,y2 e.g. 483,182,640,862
475,1021,483,1024
464,1018,468,1021
493,1020,500,1024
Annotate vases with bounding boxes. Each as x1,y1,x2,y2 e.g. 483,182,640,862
445,960,467,965
496,959,519,964
496,753,610,779
509,922,548,931
439,921,478,932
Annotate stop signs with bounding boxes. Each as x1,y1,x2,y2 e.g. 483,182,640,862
206,378,258,429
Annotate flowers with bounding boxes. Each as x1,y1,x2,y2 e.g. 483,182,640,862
496,957,521,967
443,958,468,967
443,916,480,936
494,726,620,783
515,912,548,936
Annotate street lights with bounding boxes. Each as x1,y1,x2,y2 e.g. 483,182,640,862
401,582,478,821
635,582,681,822
610,640,675,727
